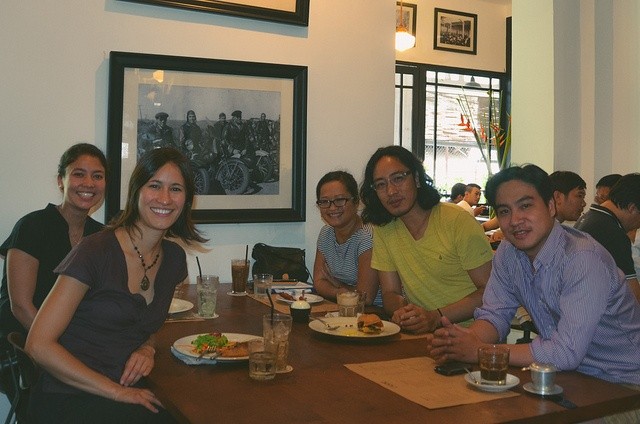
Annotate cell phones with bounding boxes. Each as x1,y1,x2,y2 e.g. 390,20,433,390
434,359,472,376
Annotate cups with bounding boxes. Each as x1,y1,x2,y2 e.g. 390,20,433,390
479,345,510,385
248,337,279,381
253,273,273,299
196,275,219,317
231,259,250,294
263,313,292,370
520,361,562,389
336,291,359,317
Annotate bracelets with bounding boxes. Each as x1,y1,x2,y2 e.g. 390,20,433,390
435,307,444,318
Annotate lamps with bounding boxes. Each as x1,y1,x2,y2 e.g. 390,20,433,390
465,76,480,88
395,2,415,51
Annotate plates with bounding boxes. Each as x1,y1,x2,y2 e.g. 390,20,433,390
173,332,264,361
308,317,401,338
523,381,564,398
464,371,520,392
276,294,324,304
167,297,194,314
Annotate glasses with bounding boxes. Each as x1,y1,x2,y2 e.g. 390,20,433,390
316,196,354,209
370,170,414,192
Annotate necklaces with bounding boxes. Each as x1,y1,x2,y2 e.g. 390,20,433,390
134,245,160,291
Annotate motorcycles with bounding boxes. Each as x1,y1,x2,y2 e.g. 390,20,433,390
190,138,250,194
139,138,212,194
228,141,273,182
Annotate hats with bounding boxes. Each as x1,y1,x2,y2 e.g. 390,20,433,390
155,113,169,119
232,112,242,116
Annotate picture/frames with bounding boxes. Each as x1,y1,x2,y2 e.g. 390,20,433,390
119,0,309,28
397,2,417,48
433,6,477,54
105,52,307,225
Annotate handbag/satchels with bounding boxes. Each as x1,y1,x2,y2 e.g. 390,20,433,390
252,243,314,283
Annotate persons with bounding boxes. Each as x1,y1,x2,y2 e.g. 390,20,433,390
359,145,495,333
215,111,250,160
447,182,486,217
179,110,203,143
24,148,171,424
480,215,504,252
428,165,639,423
573,172,640,306
249,112,280,150
549,170,586,223
0,143,105,423
142,110,174,141
312,170,384,307
595,174,640,281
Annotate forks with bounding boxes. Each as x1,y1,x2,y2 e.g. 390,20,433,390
312,316,340,330
196,344,217,361
